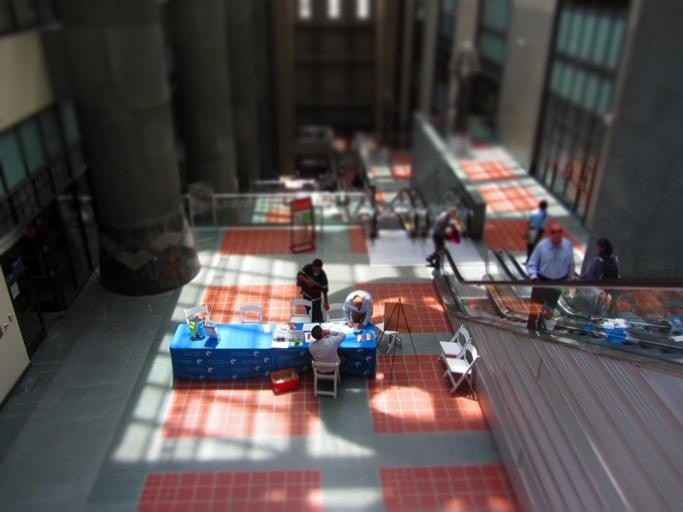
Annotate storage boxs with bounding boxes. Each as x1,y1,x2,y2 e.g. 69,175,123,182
269,368,301,394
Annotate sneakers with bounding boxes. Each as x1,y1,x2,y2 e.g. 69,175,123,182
425,256,435,266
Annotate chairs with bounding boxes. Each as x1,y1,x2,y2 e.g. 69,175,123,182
437,324,480,394
311,360,340,399
238,305,263,324
183,302,210,324
290,299,313,324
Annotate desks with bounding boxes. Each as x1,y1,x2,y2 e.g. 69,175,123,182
169,322,377,381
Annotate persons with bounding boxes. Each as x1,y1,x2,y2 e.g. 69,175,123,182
296,257,330,323
342,290,374,330
317,164,338,192
307,326,349,386
426,206,457,269
574,237,623,339
521,200,549,265
527,220,576,338
